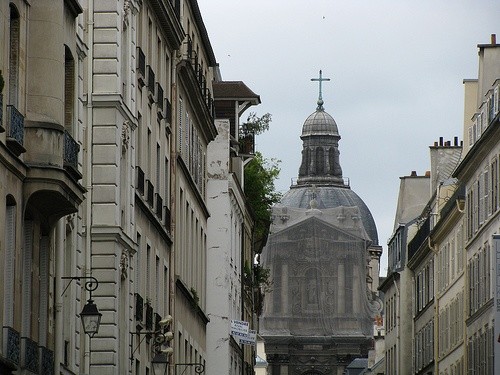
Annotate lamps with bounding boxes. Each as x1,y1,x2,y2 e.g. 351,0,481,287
52,275,102,339
